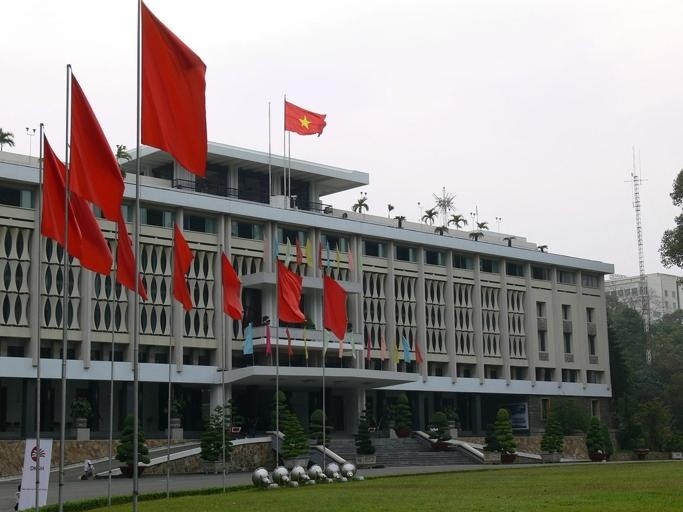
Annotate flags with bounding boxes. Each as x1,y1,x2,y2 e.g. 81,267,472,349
276,263,306,326
172,224,194,312
68,72,123,227
221,251,241,321
238,321,425,366
271,235,355,274
115,211,148,304
325,272,348,340
138,2,208,181
39,134,111,276
283,99,327,139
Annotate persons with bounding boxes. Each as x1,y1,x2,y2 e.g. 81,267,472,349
82,454,97,480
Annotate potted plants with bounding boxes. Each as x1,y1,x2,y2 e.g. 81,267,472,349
309,409,333,450
117,413,150,477
482,408,516,465
199,405,233,475
280,413,311,470
396,391,412,439
585,413,610,462
442,405,460,438
427,411,450,452
70,395,93,440
163,399,188,440
632,404,650,461
539,400,564,465
351,408,378,470
